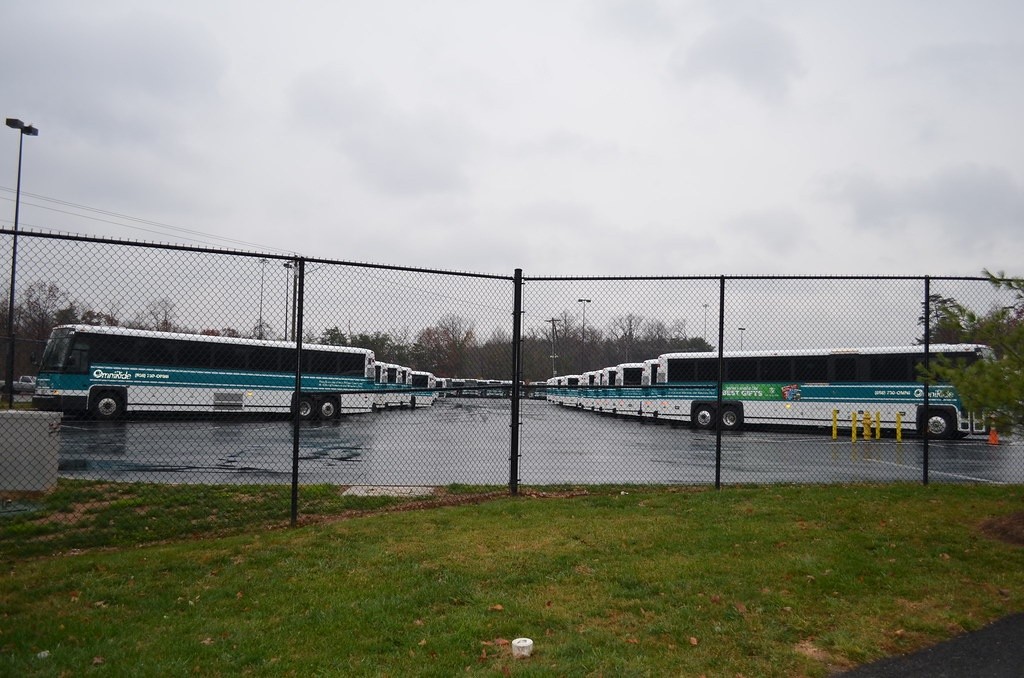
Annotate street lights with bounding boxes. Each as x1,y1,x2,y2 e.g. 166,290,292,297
260,257,273,337
703,303,708,348
5,117,44,411
578,298,591,374
738,327,748,352
283,262,293,340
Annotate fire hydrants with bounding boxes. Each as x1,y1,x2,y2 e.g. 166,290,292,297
860,411,873,442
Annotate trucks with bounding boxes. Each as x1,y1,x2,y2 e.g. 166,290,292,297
0,375,37,395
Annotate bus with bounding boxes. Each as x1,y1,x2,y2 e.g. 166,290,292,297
39,323,380,421
374,360,454,409
460,379,526,399
655,344,1000,441
614,362,642,421
545,366,617,417
640,359,661,426
528,381,547,400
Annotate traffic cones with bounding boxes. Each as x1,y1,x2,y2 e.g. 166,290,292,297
986,422,1005,446
